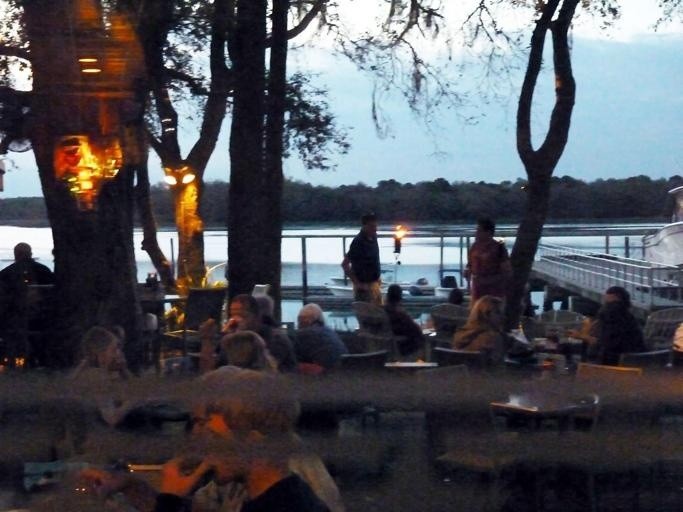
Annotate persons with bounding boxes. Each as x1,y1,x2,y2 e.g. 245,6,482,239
342,213,382,303
446,285,644,367
1,244,349,511
382,282,422,336
463,217,514,311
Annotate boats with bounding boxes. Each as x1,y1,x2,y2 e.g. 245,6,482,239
324,267,411,298
641,185,683,283
